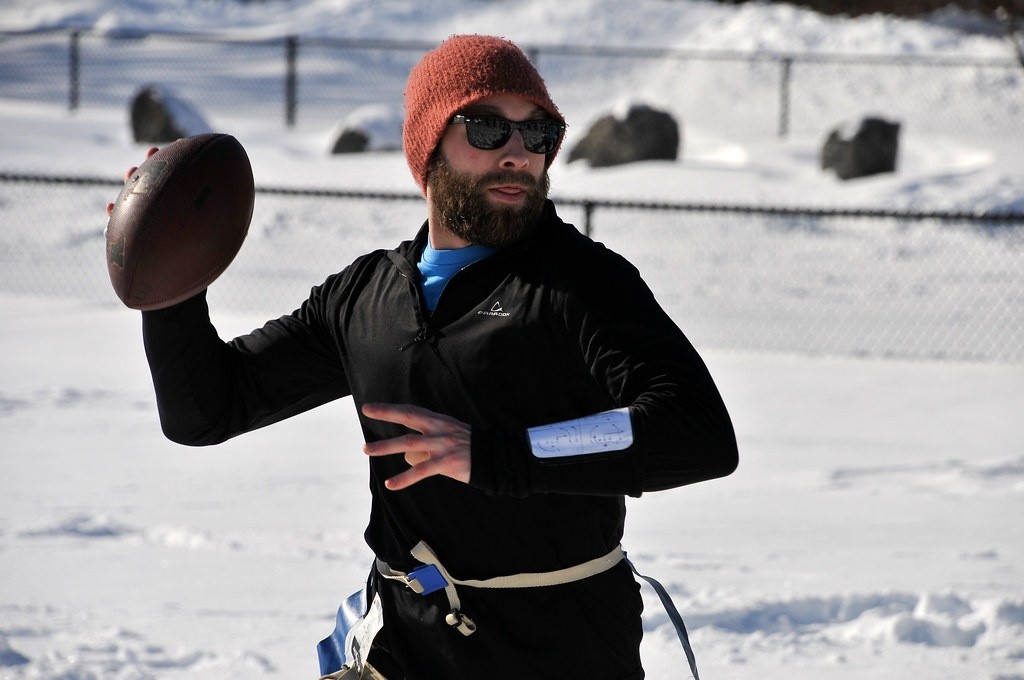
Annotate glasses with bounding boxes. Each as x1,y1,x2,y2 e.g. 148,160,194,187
452,111,569,154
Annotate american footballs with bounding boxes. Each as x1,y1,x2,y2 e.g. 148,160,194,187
105,132,256,312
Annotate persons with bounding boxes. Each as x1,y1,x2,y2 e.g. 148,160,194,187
109,35,740,680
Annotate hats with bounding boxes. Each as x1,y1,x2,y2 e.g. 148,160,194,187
401,35,567,198
